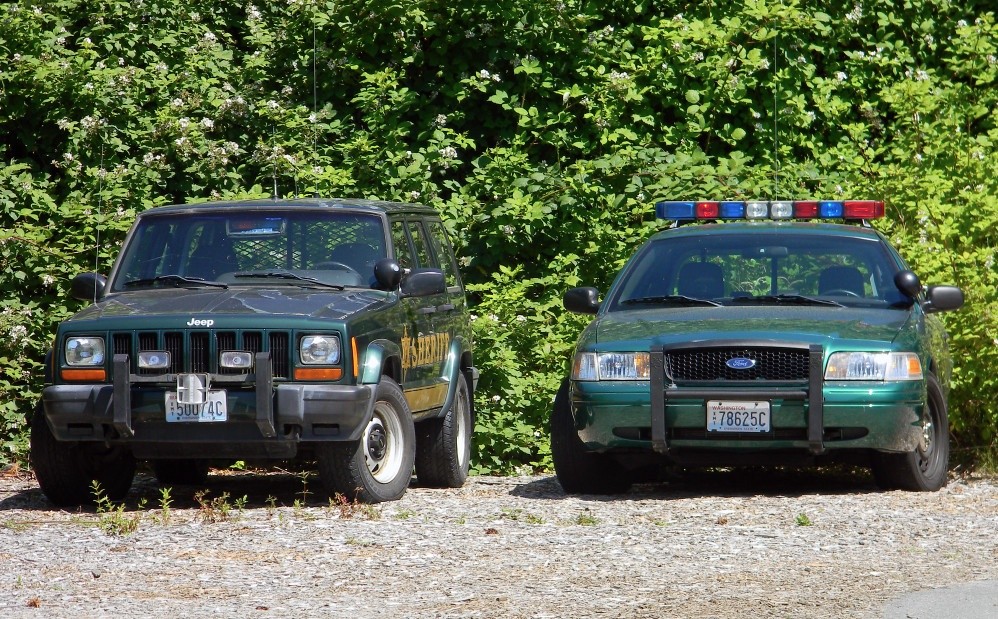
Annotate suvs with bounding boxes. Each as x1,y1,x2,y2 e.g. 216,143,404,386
30,197,479,510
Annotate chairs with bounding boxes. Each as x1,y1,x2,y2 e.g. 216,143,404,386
187,247,241,281
818,266,866,299
332,243,382,288
677,260,727,300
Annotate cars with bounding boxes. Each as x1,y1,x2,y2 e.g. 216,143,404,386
548,198,967,493
